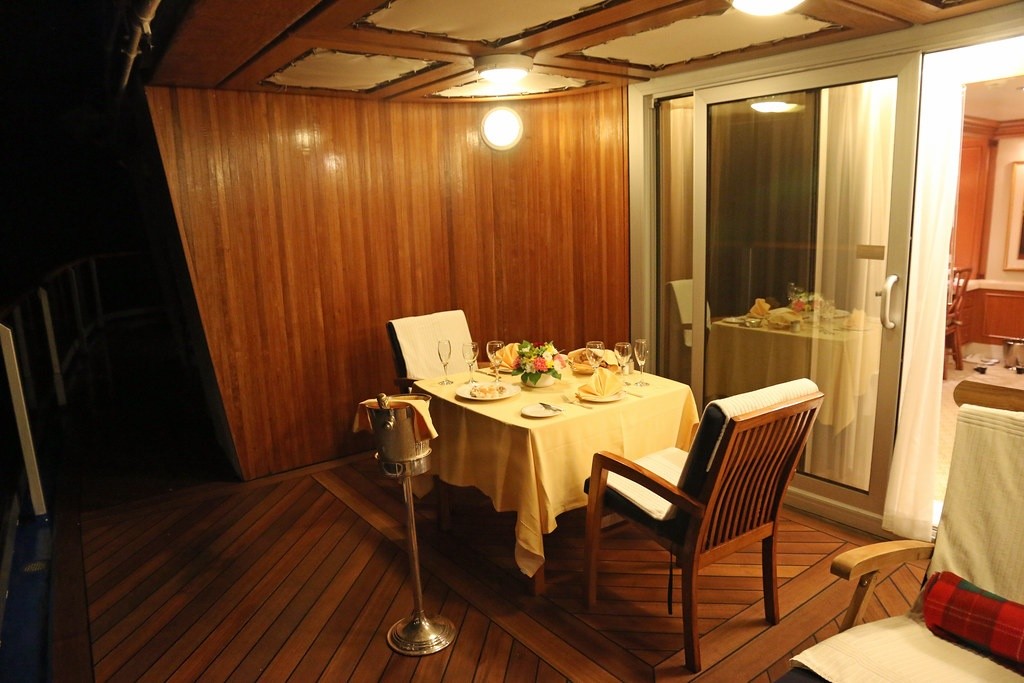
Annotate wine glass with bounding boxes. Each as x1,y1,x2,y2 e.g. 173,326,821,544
435,339,453,385
586,339,649,381
486,340,504,382
462,340,479,385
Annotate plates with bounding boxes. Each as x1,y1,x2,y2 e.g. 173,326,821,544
456,381,521,400
521,403,564,418
577,388,622,403
743,303,878,331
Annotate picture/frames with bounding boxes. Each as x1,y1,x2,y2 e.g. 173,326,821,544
1004,161,1024,271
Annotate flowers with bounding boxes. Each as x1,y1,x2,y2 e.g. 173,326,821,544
511,339,561,384
787,283,824,313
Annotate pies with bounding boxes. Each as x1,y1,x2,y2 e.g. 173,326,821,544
574,349,608,368
470,385,507,398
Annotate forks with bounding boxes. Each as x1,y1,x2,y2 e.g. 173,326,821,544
561,395,592,409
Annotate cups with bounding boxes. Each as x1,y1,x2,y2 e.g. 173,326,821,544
786,282,807,303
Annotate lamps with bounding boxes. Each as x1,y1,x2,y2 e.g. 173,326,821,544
724,0,806,15
479,106,529,156
473,53,534,86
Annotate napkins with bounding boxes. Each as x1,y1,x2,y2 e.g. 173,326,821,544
847,310,866,327
749,298,801,327
496,343,624,399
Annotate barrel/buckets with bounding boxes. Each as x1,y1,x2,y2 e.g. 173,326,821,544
1003,338,1024,369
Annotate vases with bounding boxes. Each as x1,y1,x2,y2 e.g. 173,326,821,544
521,372,552,389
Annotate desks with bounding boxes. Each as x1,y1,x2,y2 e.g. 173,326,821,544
703,307,885,482
412,353,700,599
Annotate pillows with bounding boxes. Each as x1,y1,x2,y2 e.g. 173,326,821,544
922,571,1024,667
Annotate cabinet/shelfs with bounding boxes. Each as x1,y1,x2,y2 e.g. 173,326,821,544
945,288,1024,350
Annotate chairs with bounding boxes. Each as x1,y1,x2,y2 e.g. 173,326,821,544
770,379,1024,683
664,281,711,384
583,377,826,673
943,268,974,380
385,308,479,395
